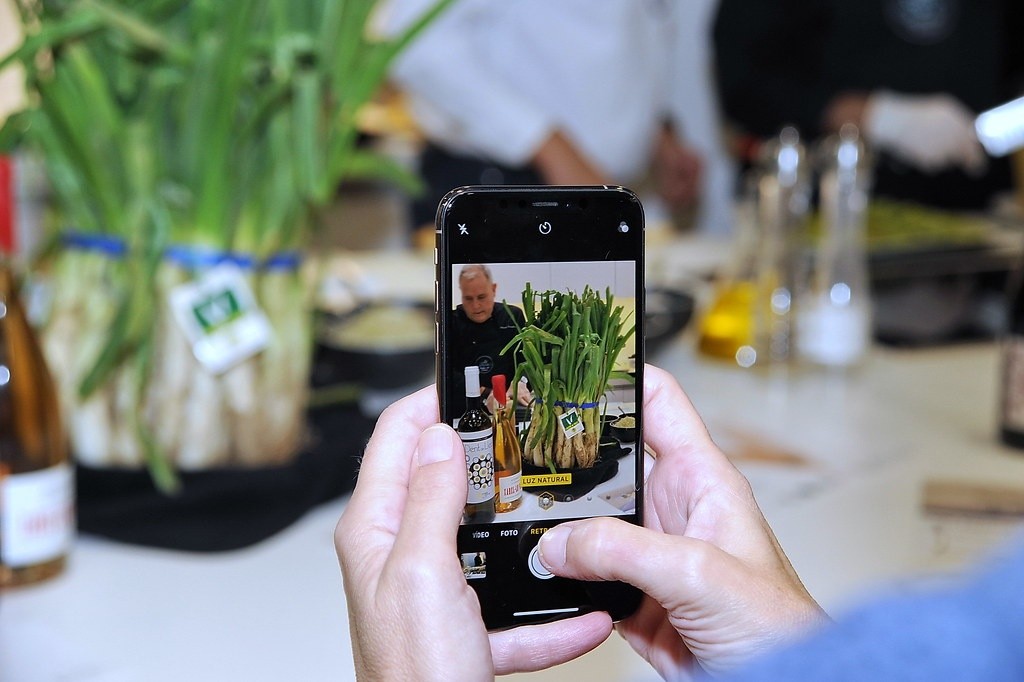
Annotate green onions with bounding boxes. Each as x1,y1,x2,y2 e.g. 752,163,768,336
0,0,450,496
499,284,636,474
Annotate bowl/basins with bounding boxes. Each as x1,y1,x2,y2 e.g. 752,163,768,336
643,281,696,354
600,413,635,443
314,298,435,387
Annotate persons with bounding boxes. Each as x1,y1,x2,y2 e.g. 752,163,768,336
332,363,1024,682
712,0,1024,210
451,265,533,410
380,1,727,243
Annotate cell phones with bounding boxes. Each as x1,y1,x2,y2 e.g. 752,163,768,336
434,184,646,635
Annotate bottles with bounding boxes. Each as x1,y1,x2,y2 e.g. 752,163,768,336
457,366,495,524
490,375,523,514
0,154,74,591
699,136,873,367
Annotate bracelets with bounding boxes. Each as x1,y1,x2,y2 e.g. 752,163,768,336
480,387,491,403
